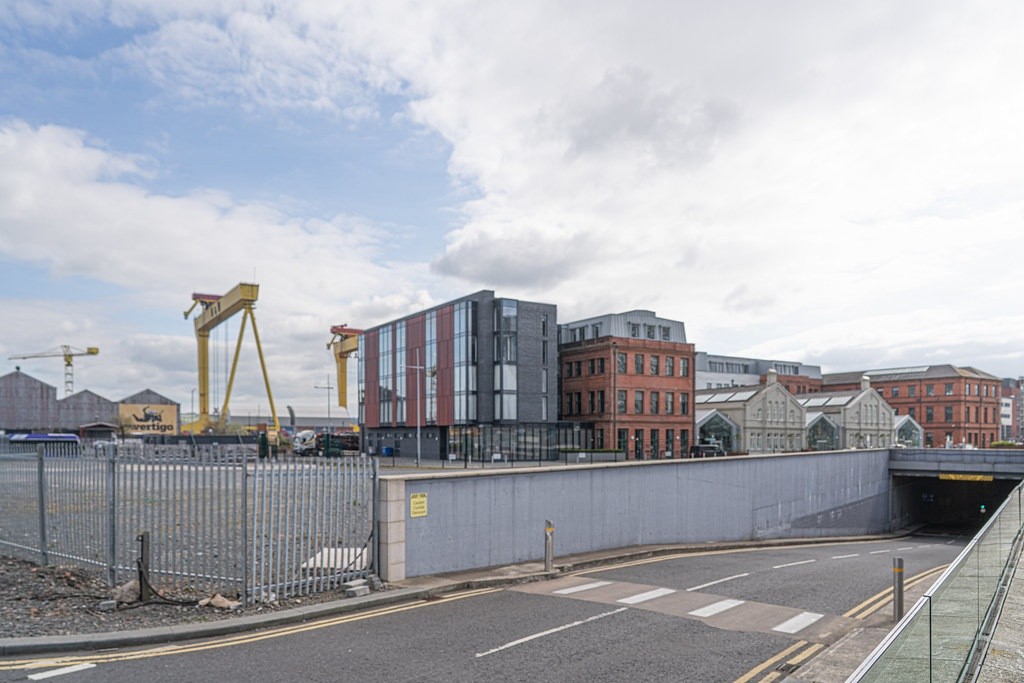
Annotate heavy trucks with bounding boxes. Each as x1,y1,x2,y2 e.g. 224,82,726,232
291,429,360,457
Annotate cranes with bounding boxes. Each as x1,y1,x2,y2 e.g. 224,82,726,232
325,325,364,409
6,343,100,435
182,282,283,440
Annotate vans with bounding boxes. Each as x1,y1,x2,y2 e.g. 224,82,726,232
689,444,727,459
